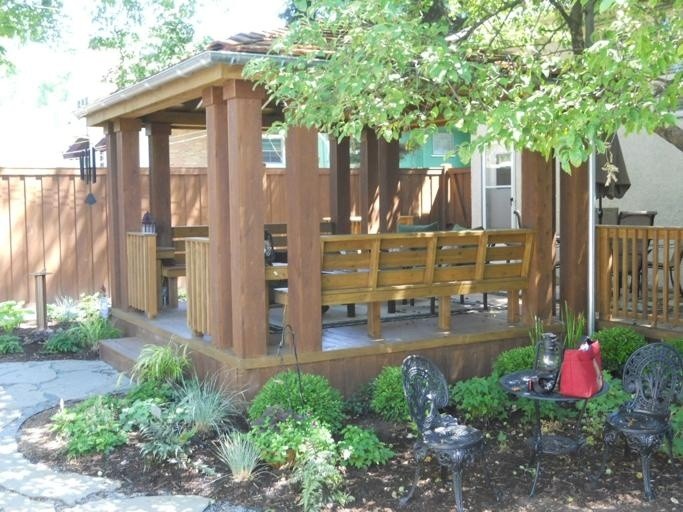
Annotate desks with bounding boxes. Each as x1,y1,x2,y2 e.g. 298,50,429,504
497,368,612,498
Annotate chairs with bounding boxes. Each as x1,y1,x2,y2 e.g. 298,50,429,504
602,340,683,502
162,218,538,342
401,352,489,510
598,207,683,288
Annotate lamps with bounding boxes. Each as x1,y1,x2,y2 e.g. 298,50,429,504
532,331,563,379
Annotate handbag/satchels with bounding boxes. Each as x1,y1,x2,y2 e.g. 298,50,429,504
559,336,603,398
